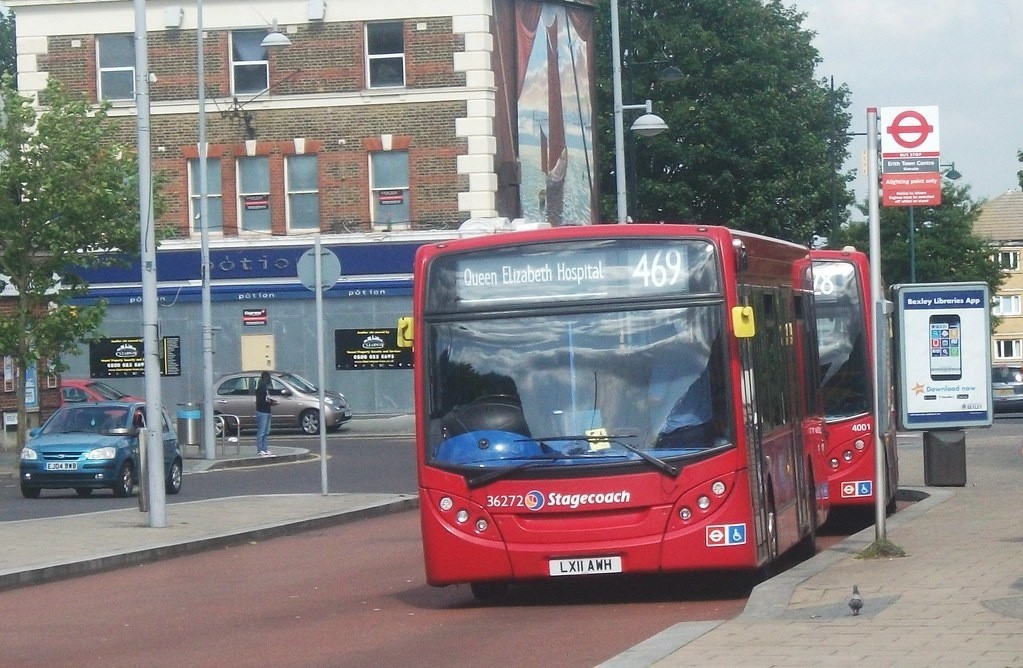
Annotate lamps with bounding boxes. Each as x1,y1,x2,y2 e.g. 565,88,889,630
259,17,294,47
622,98,669,137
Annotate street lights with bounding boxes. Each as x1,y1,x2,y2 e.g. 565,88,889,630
608,0,668,223
909,163,961,283
627,1,685,223
194,0,293,462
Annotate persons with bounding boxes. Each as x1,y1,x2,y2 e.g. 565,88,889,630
253,370,280,459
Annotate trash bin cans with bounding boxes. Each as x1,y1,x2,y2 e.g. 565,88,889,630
176,402,207,460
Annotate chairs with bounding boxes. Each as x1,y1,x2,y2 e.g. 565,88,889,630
65,411,146,436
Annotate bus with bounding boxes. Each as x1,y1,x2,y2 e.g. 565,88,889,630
412,220,828,606
803,246,900,515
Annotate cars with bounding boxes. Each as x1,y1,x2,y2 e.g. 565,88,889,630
60,378,149,425
200,370,352,436
17,399,182,498
990,362,1023,413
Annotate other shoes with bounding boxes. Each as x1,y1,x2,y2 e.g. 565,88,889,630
260,451,275,458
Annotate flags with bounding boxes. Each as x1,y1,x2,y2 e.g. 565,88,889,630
510,0,602,232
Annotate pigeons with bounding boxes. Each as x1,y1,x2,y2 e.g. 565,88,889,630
848,584,863,616
